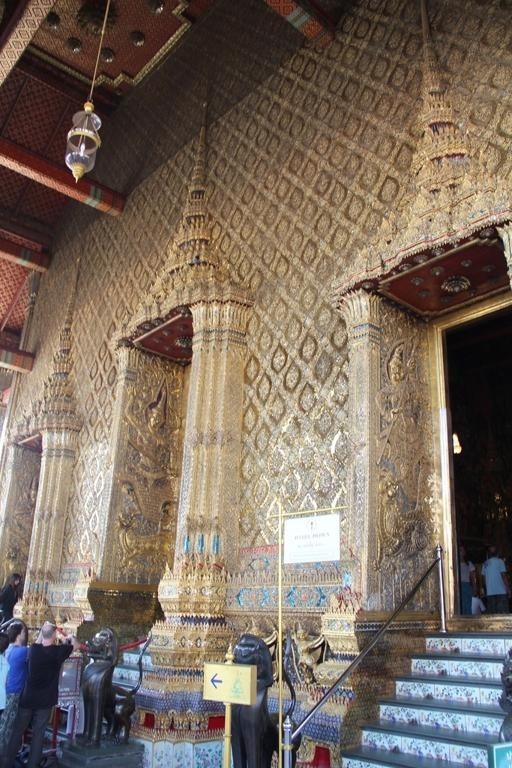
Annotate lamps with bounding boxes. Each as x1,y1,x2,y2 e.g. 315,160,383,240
64,0,112,185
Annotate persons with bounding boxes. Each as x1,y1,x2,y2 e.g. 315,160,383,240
0,574,21,621
0,632,0,713
0,621,32,759
0,621,73,768
459,544,477,614
480,545,511,613
472,592,487,615
0,633,11,716
